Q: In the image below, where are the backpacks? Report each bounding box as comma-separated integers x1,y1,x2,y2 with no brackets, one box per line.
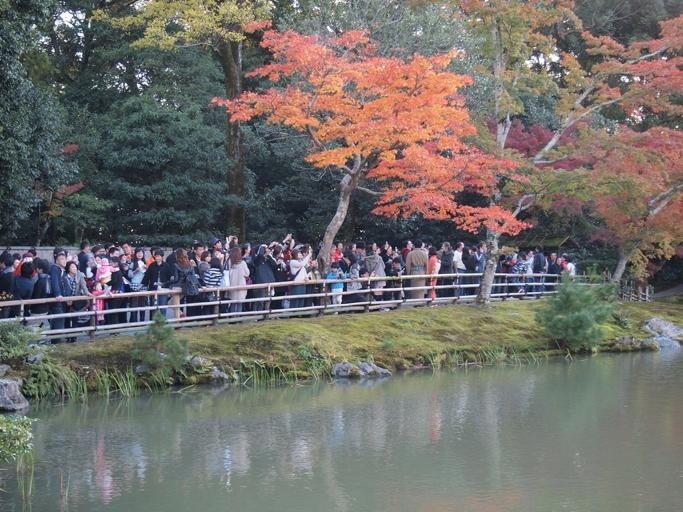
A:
174,262,199,298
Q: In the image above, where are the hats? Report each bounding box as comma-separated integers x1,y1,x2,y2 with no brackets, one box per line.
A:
291,248,302,259
207,237,221,247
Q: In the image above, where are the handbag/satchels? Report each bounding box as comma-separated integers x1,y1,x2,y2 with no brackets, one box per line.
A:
284,272,295,283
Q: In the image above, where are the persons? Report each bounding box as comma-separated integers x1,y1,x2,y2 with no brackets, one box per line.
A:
1,228,579,333
